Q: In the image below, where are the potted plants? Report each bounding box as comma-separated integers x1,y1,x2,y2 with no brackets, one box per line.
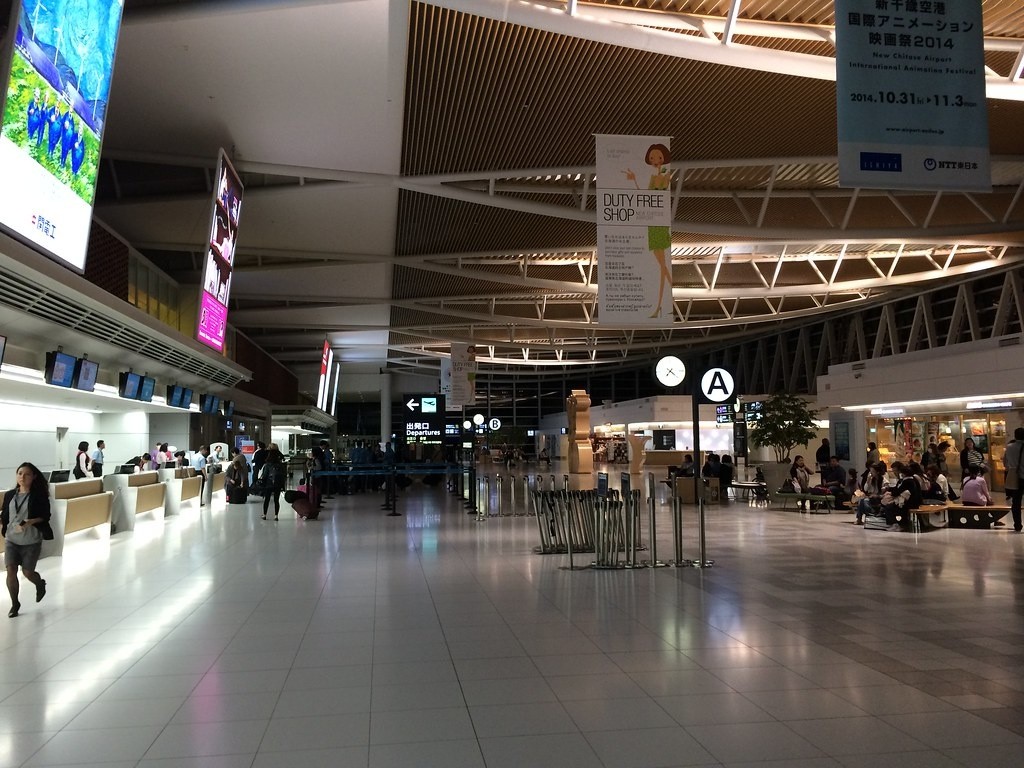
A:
755,395,821,501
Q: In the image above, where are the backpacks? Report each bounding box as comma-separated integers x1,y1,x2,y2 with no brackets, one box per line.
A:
915,474,946,504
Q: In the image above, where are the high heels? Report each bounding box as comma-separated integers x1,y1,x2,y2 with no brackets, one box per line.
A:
9,603,20,618
36,579,46,602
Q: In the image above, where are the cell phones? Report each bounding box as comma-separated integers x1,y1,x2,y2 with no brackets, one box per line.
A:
19,520,26,526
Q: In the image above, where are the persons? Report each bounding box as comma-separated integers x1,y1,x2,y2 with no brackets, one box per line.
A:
90,440,105,477
790,455,814,510
150,442,172,471
853,460,949,532
0,462,54,618
841,468,861,494
823,455,847,503
224,447,248,501
252,442,285,521
540,448,552,466
126,453,151,471
211,445,222,463
499,446,525,468
702,454,735,485
816,438,830,484
921,441,960,501
961,463,1005,526
1003,428,1024,531
681,454,693,474
306,440,332,504
867,442,879,462
192,445,208,506
349,440,395,493
73,441,91,479
960,438,984,477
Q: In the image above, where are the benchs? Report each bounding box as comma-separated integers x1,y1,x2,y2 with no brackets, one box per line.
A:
772,492,1024,532
729,478,766,489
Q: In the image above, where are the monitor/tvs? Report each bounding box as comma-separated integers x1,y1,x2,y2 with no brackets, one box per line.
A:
166,461,176,468
0,335,7,368
120,464,135,474
200,394,219,415
45,351,99,392
224,400,235,416
119,372,156,402
166,384,193,409
50,470,70,483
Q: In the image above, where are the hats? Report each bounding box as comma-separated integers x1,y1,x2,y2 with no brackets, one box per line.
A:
938,442,950,450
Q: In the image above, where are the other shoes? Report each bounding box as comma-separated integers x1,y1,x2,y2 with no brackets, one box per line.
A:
261,515,266,520
801,505,807,510
952,497,960,501
226,496,230,502
895,525,906,532
887,524,897,530
853,521,863,524
994,522,1005,526
274,518,278,521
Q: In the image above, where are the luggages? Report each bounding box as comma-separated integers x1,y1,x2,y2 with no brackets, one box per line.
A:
281,490,319,521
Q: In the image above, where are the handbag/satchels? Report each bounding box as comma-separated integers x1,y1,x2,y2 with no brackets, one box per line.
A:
256,479,266,487
779,478,801,494
809,485,833,495
230,485,247,504
